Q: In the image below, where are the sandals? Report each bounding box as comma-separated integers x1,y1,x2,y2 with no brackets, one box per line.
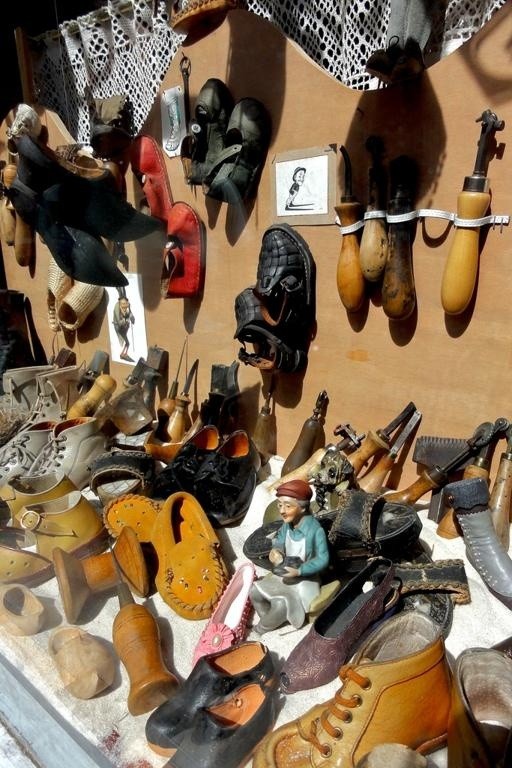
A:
276,555,400,691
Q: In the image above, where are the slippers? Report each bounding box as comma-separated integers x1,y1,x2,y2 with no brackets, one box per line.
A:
372,541,471,643
242,492,421,566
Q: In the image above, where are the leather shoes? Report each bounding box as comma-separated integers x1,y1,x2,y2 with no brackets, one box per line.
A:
255,222,318,345
166,682,274,766
145,640,275,756
238,609,456,760
232,290,281,377
1,367,263,623
2,77,273,331
445,644,512,760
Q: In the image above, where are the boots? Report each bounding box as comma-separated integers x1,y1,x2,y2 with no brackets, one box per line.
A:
449,475,512,603
366,0,446,88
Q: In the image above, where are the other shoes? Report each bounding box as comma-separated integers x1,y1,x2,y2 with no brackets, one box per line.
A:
49,626,115,700
190,560,259,668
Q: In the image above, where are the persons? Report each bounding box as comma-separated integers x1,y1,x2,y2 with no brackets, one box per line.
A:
248,480,330,640
112,286,135,362
284,167,314,210
